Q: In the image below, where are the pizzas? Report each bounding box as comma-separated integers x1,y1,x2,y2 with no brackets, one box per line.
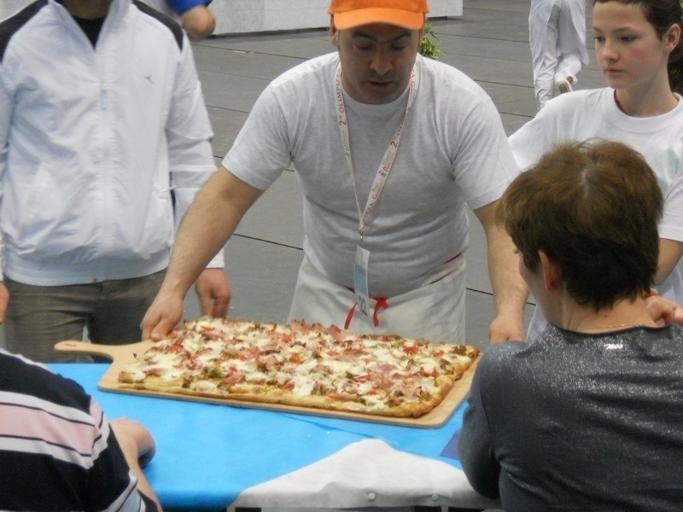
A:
118,315,480,418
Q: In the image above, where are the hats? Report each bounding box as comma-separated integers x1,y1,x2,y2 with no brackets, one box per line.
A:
327,0,429,32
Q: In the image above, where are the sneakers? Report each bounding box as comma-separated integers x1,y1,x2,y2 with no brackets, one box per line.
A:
557,78,574,93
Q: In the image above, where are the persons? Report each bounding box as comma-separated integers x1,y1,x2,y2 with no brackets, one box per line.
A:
520,0,590,113
136,0,528,349
0,1,235,370
2,272,163,512
448,136,680,509
495,1,681,349
